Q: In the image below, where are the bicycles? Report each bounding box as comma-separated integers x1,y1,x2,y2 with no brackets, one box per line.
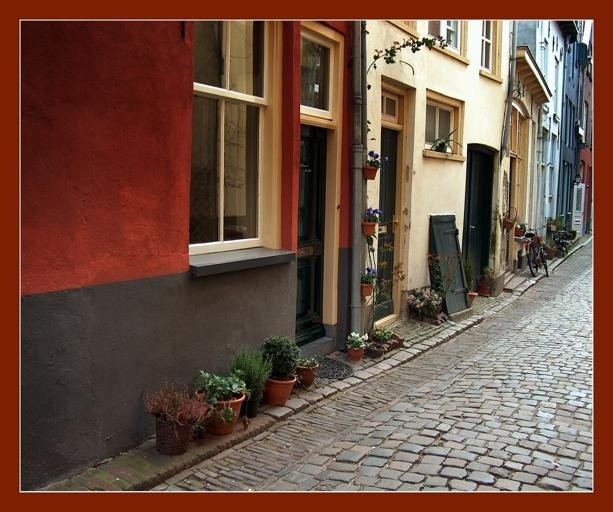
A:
557,223,570,258
523,225,549,277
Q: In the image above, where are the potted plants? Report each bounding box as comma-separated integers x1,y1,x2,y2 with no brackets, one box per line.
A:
464,263,478,305
430,128,463,155
548,209,565,231
143,337,319,455
546,240,556,260
478,264,494,296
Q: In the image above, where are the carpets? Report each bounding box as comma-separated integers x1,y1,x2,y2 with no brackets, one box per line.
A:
313,353,354,379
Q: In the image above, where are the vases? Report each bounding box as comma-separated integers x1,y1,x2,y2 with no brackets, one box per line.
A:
515,228,525,236
364,167,378,180
348,347,364,360
362,222,377,236
361,283,372,296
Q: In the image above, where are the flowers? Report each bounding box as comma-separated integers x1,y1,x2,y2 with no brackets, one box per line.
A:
361,267,375,284
515,224,526,230
408,288,442,308
362,208,382,222
364,150,389,169
345,332,369,350
